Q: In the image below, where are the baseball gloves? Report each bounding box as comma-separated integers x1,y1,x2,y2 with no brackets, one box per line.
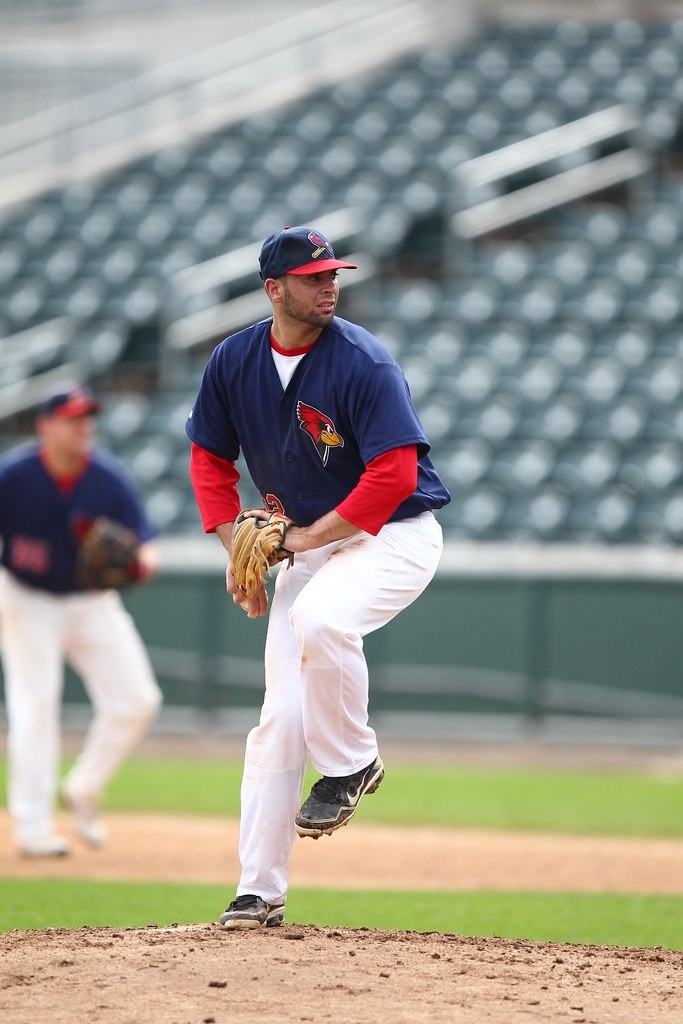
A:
229,507,295,620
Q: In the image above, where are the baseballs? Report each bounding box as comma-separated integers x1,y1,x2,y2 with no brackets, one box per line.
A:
239,587,269,613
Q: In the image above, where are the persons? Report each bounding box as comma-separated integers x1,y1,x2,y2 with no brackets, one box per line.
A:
185,226,452,929
0,388,164,859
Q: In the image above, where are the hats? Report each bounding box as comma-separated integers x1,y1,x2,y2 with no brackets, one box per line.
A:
258,225,357,281
37,381,98,419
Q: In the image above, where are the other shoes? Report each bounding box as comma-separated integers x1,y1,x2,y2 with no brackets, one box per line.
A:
18,836,68,857
56,784,101,846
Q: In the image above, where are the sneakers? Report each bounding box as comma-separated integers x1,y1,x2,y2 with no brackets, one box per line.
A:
293,754,384,838
219,895,285,930
